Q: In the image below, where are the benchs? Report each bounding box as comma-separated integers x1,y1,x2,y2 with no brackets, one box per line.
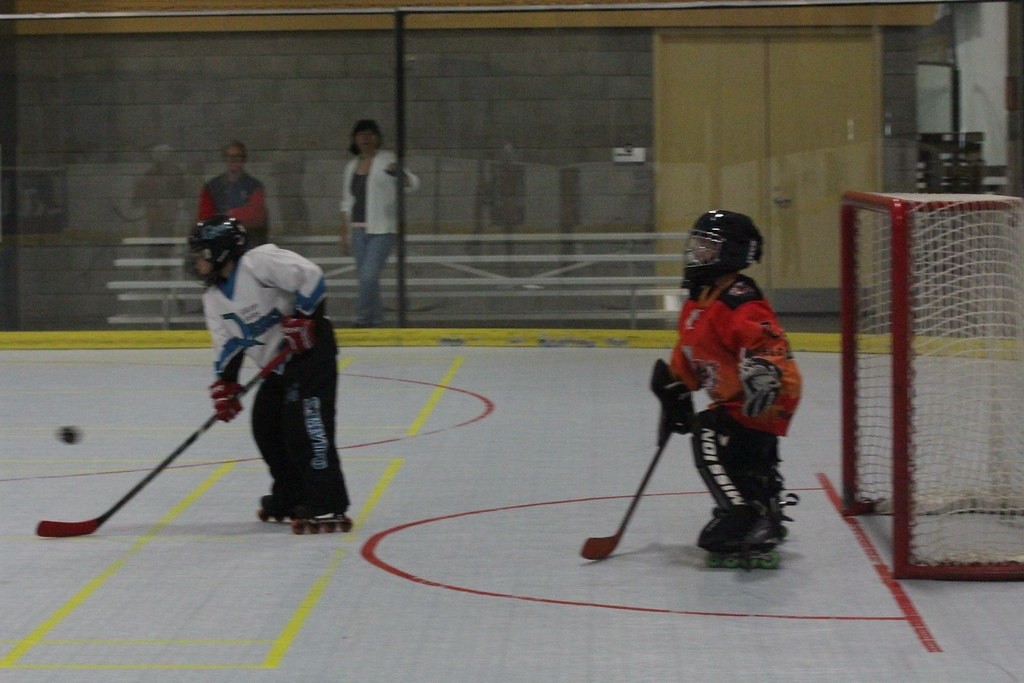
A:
110,229,690,321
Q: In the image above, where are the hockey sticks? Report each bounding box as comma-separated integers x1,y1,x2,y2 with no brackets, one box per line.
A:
580,415,677,561
36,346,291,538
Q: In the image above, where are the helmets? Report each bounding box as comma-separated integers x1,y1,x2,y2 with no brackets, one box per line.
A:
684,211,763,288
184,215,247,287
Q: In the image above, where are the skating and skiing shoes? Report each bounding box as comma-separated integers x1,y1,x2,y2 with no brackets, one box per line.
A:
288,503,352,535
698,496,788,570
257,485,294,522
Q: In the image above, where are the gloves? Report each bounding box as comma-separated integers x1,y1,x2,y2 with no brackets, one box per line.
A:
281,316,316,354
650,360,700,436
738,357,784,419
210,380,242,422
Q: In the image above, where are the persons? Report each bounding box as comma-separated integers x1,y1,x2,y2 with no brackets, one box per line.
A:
340,119,419,328
199,142,264,246
191,218,354,533
652,210,803,568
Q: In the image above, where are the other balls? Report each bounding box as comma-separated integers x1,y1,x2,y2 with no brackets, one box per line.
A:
62,427,76,444
